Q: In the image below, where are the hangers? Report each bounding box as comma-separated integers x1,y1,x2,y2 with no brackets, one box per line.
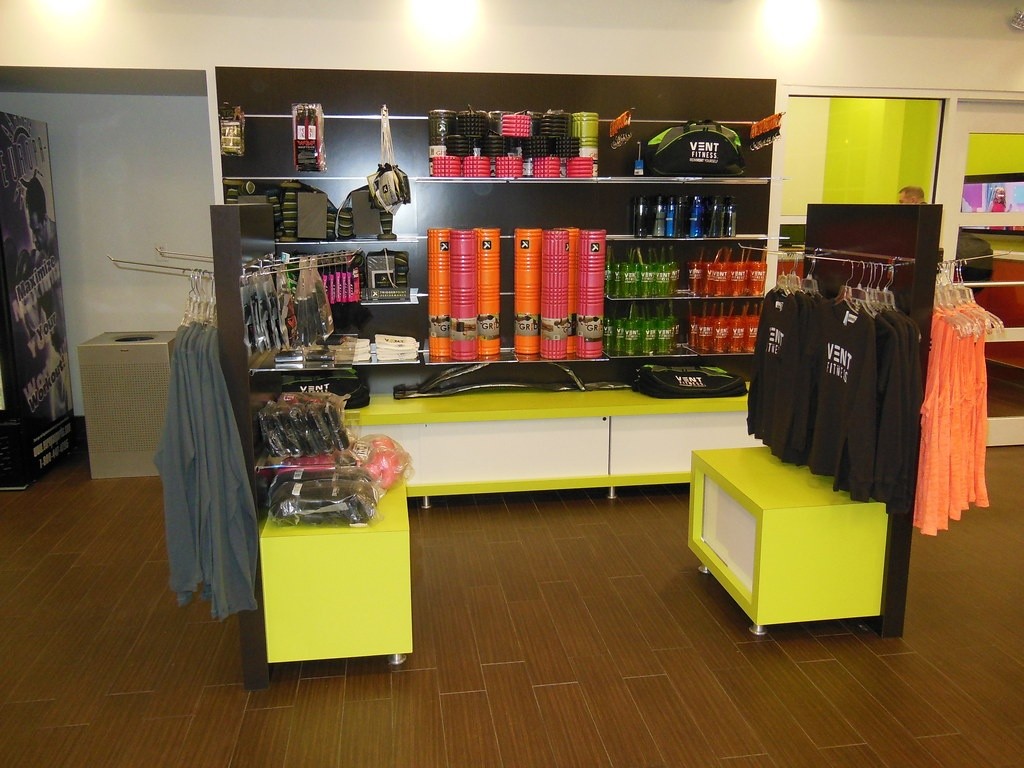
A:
934,258,1005,339
178,269,218,330
773,254,819,297
831,260,901,320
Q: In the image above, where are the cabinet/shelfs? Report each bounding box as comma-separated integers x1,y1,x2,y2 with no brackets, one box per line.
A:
688,446,889,635
259,476,414,664
216,65,776,509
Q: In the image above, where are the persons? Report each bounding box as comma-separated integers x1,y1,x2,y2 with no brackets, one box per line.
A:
23,177,74,354
898,185,925,204
983,187,1012,230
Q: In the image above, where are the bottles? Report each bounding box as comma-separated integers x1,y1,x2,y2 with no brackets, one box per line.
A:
635,195,737,237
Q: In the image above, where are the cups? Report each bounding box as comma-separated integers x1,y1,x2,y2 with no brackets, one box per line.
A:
604,317,760,354
605,259,767,297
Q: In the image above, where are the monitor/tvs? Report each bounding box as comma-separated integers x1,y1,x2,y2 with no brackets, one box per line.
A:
960,172,1024,236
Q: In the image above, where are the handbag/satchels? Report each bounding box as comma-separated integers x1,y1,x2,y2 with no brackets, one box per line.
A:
281,367,371,410
631,360,749,399
643,119,747,176
